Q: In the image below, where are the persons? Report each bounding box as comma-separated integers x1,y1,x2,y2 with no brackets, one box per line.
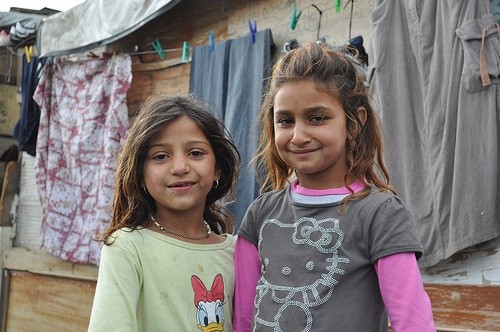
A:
233,41,438,332
86,94,237,332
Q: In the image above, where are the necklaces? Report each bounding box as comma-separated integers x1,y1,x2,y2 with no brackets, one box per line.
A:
150,214,212,240
